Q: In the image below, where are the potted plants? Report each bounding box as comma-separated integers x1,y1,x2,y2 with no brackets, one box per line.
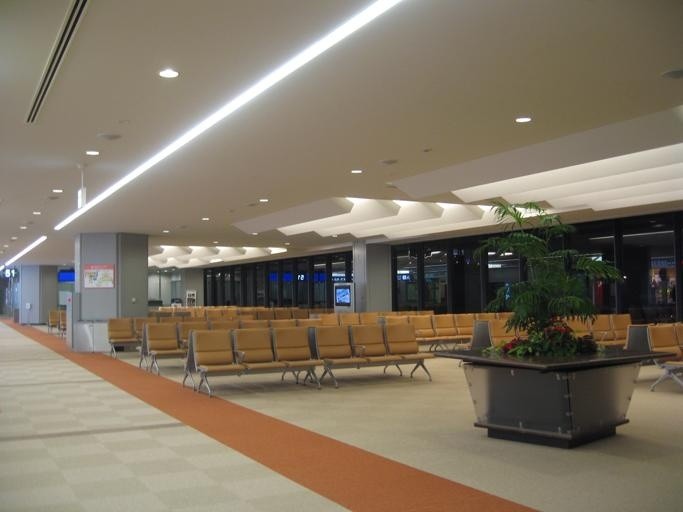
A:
433,200,680,448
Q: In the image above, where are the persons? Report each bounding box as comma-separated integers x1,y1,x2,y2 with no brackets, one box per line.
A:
651,268,673,304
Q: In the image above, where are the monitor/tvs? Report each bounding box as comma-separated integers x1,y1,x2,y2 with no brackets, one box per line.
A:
57,269,75,282
335,285,351,306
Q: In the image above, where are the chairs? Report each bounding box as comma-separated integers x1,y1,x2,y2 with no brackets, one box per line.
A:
48,306,683,397
